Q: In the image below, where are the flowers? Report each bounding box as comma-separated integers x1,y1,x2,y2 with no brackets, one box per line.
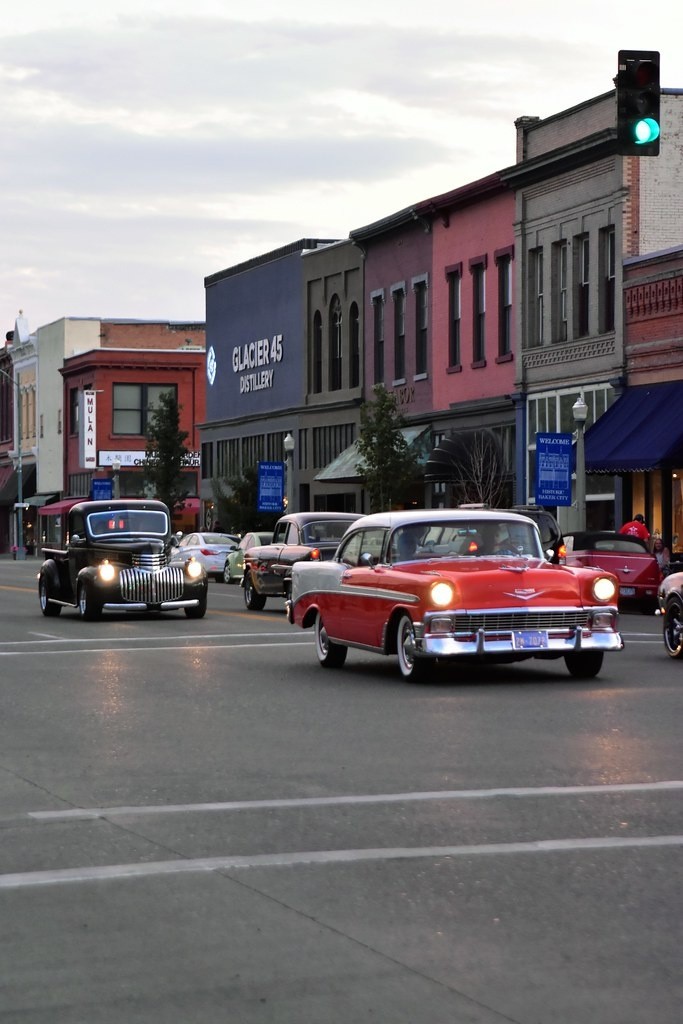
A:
10,546,27,552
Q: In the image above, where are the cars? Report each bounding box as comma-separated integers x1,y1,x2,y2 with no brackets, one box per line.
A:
283,508,625,681
37,497,209,622
170,530,243,584
239,510,395,611
657,572,683,658
221,531,315,588
562,529,665,615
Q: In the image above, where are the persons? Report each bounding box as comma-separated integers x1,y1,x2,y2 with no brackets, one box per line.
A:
213,521,225,533
397,533,418,561
618,514,670,567
463,523,515,556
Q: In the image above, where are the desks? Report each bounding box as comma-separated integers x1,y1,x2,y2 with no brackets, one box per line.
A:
665,562,683,575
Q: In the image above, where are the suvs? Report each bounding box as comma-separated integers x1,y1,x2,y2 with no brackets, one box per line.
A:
457,501,566,566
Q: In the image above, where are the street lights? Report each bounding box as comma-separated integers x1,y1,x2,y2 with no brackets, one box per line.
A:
571,394,589,531
283,434,297,515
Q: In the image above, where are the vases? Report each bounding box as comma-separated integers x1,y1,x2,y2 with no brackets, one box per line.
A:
12,550,29,560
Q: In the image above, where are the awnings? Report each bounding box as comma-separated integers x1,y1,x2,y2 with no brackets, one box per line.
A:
0,464,38,506
25,495,57,506
113,498,200,515
562,378,683,474
312,423,438,485
38,498,89,515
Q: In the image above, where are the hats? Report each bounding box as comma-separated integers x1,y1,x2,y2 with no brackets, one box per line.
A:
635,515,643,523
